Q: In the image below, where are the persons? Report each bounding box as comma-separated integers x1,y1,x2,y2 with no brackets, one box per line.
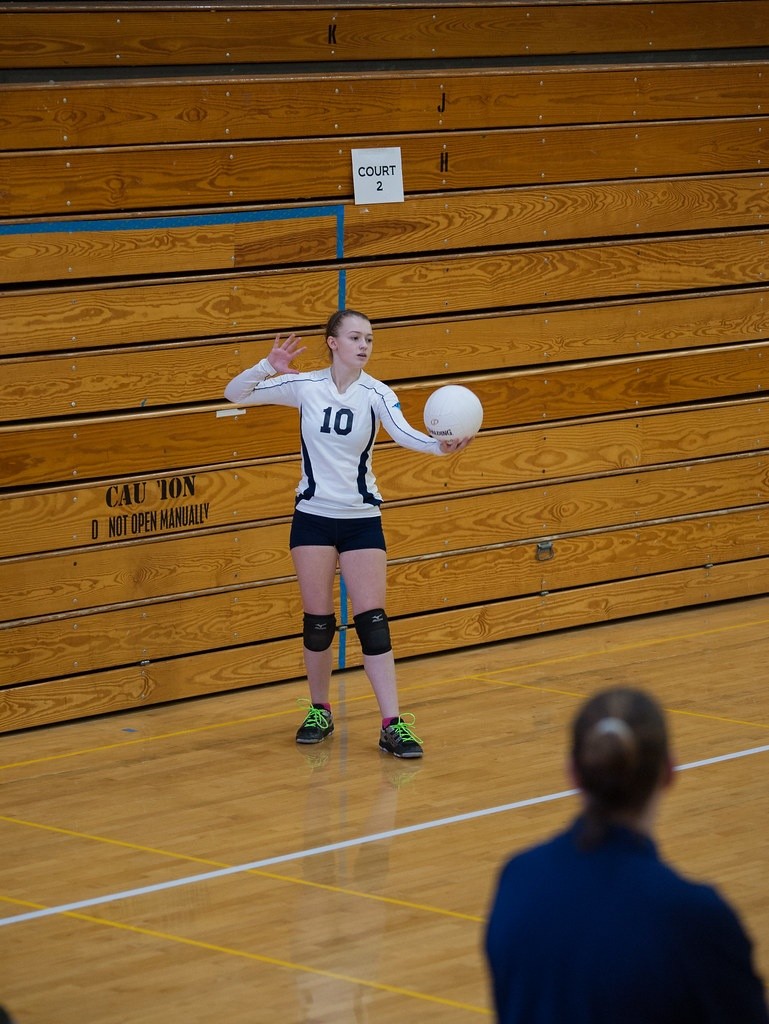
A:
222,307,478,762
479,683,767,1024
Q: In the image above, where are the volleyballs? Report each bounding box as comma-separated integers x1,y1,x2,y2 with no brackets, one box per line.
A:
423,385,484,446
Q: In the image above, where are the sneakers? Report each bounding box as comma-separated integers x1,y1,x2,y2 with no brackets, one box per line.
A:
296,708,335,744
379,717,423,759
